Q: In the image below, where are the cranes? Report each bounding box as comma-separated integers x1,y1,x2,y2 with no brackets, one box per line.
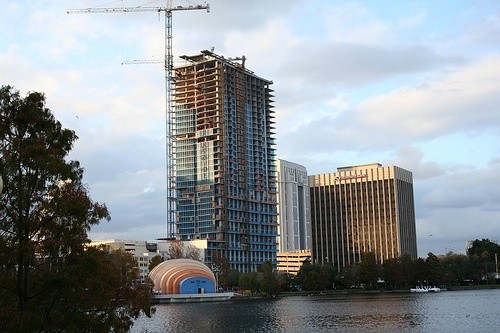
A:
67,0,210,245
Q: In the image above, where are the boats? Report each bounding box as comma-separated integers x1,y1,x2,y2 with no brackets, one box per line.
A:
410,279,440,293
